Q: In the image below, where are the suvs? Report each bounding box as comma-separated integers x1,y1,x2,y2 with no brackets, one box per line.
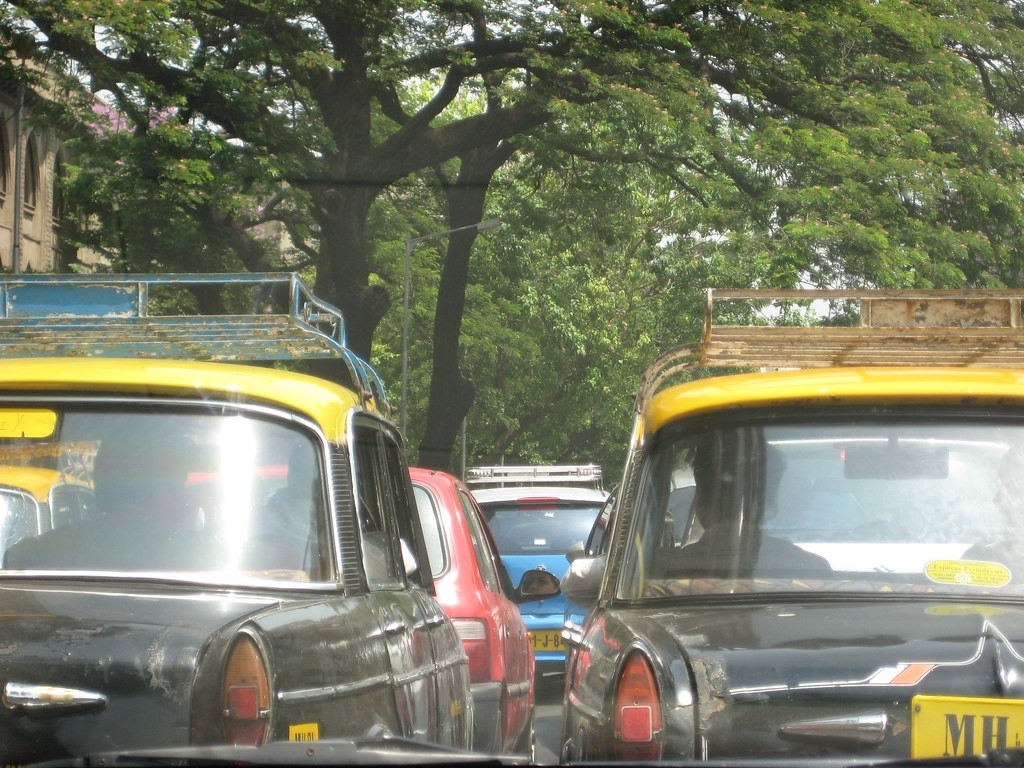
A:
567,288,1024,768
0,270,478,753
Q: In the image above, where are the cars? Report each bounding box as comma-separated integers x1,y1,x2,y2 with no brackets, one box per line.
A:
181,466,563,768
0,463,99,563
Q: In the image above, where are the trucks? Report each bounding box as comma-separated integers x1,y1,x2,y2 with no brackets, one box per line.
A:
462,463,622,702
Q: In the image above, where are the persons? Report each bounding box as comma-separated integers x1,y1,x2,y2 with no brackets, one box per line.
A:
523,571,558,594
251,440,417,578
962,438,1024,584
559,432,831,592
2,422,200,570
